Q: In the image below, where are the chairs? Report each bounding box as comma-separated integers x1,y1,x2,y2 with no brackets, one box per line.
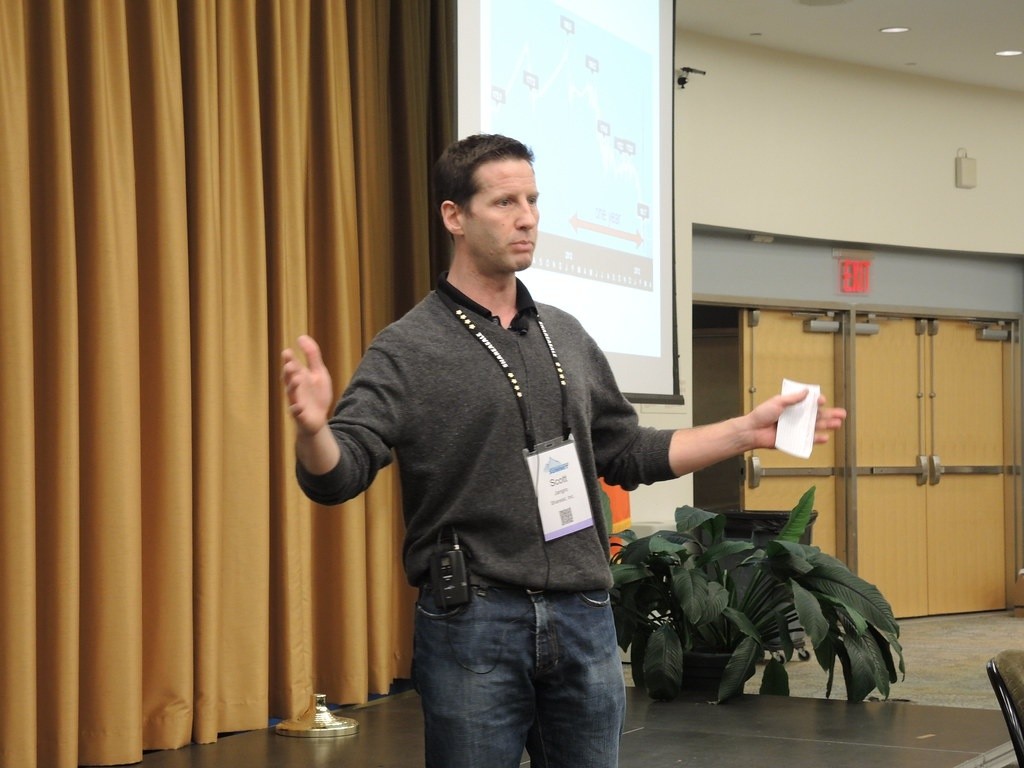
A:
987,649,1024,768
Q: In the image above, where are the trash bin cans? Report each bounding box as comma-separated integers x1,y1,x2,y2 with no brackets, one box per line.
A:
724,510,818,650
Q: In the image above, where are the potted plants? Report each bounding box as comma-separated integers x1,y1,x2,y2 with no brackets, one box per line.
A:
610,485,907,705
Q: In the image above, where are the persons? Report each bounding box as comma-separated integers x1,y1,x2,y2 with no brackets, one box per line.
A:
280,134,848,768
